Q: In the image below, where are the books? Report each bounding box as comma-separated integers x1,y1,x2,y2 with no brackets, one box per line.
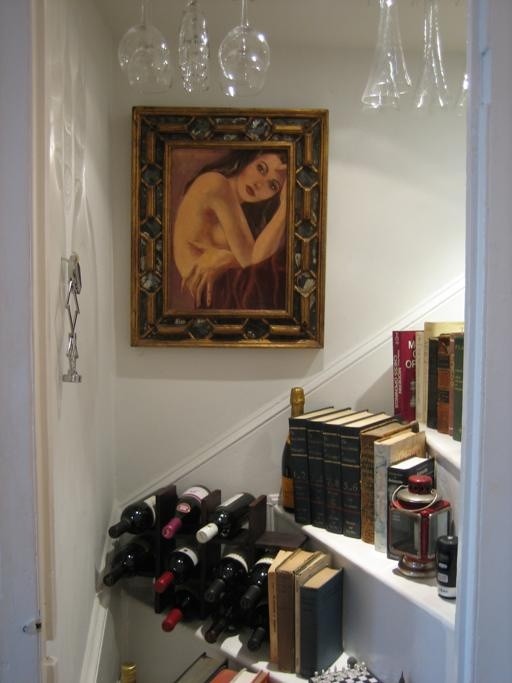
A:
374,428,427,553
360,420,419,544
307,408,370,529
386,454,435,560
268,549,294,670
275,548,332,674
342,413,400,538
289,405,351,523
300,566,344,681
322,411,385,533
393,323,462,441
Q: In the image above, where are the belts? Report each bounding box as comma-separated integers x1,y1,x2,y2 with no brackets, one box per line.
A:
216,1,271,99
117,1,172,93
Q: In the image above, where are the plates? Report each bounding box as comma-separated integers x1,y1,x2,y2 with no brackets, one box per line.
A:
118,402,465,682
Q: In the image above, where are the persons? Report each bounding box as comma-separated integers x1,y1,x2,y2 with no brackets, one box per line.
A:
172,150,287,312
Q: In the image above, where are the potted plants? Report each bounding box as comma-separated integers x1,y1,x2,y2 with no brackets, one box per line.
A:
125,103,328,355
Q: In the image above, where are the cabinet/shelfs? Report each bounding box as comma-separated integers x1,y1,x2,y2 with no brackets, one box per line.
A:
101,485,277,682
281,387,306,514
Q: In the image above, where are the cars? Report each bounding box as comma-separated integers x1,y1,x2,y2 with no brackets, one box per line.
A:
359,0,402,111
176,1,212,94
411,1,452,113
